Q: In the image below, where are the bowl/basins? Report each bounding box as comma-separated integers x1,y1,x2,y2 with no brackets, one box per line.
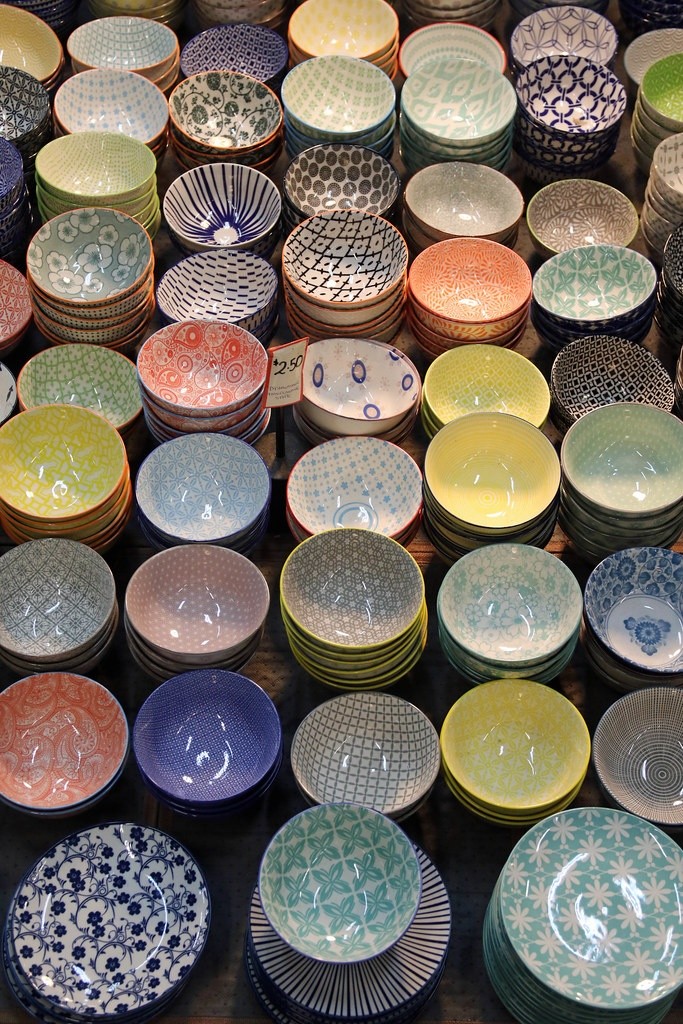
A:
0,0,683,962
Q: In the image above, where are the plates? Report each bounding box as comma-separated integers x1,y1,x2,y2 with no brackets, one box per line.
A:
0,822,211,1024
246,841,451,1024
483,806,683,1024
